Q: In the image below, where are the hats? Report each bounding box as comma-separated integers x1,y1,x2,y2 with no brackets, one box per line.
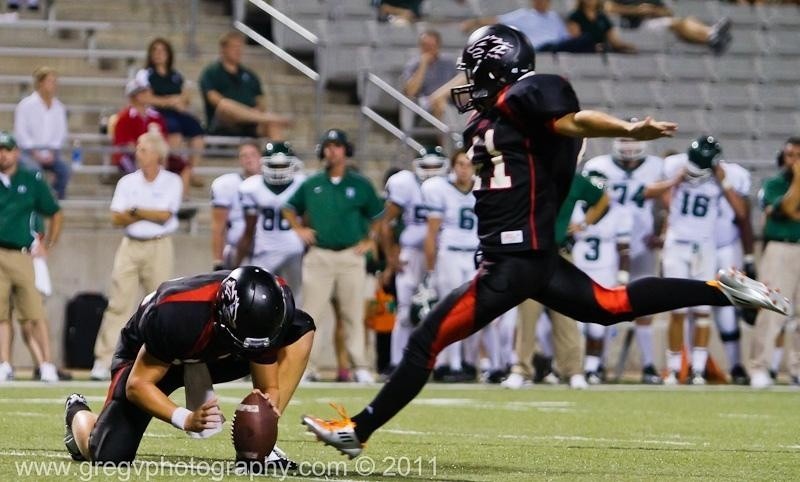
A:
316,128,354,158
0,133,25,152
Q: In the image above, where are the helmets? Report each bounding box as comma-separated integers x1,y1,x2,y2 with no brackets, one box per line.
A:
412,148,449,184
215,267,285,364
610,115,722,188
260,141,297,184
451,23,534,114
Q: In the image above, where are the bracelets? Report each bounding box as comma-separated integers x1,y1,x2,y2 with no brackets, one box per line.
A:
170,406,193,431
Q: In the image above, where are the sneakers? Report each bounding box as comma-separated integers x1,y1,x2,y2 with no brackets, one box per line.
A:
64,393,93,461
0,359,111,383
716,267,793,315
300,401,366,460
293,349,797,387
236,446,297,470
710,17,731,58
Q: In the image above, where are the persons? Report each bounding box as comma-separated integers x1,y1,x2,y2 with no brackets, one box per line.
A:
60,262,319,473
301,24,795,463
1,1,799,385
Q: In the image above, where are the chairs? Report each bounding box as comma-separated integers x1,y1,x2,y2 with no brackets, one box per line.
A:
271,1,800,239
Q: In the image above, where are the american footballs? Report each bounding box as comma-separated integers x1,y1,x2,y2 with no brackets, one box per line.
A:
231,393,278,472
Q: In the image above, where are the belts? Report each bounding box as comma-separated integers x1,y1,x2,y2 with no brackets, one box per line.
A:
767,236,800,244
0,243,30,253
447,246,477,253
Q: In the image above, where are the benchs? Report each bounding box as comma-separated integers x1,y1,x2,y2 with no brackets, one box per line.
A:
0,1,419,236
